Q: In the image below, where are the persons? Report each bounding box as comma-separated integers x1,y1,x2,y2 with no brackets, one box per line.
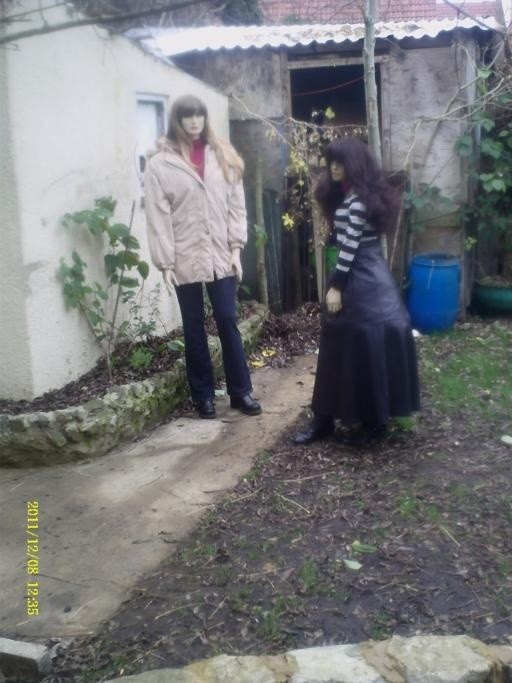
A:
142,95,262,416
295,138,421,444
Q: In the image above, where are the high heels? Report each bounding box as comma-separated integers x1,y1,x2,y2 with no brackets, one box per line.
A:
197,400,216,418
230,394,262,415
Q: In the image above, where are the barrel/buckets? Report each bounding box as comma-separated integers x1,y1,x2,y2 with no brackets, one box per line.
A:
407,249,462,337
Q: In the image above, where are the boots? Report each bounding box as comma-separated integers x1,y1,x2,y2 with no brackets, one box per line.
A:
294,416,335,445
345,420,387,446
